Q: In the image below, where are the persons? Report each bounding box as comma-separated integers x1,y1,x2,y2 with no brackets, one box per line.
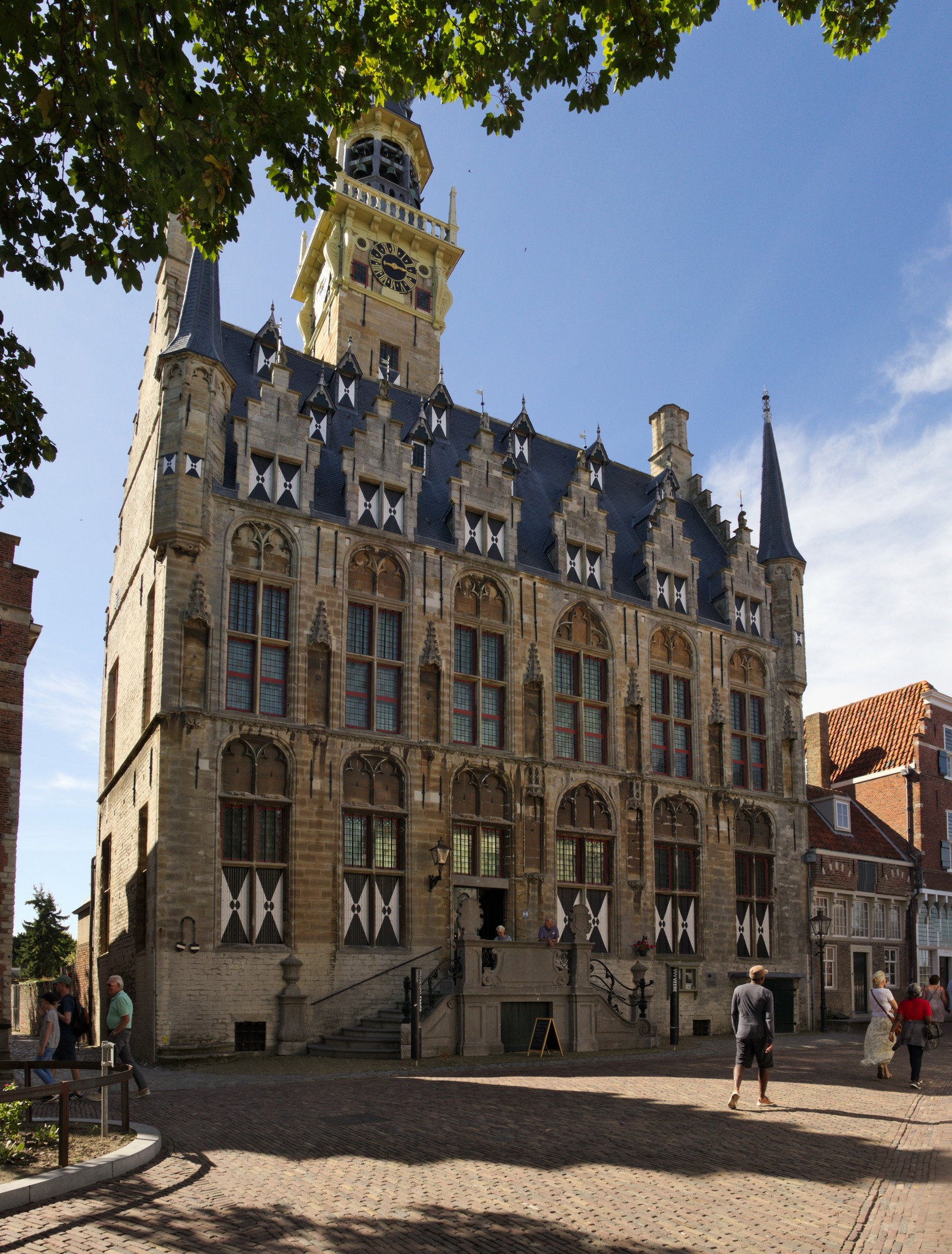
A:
922,974,949,1046
860,970,898,1079
36,974,83,1101
494,925,512,940
728,965,774,1110
538,915,559,947
34,992,59,1105
945,980,952,1014
888,983,932,1089
87,975,150,1101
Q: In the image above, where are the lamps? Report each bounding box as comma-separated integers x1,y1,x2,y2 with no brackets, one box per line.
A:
429,837,452,892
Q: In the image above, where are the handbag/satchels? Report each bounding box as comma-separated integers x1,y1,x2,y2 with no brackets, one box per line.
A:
890,1016,902,1036
926,1021,944,1050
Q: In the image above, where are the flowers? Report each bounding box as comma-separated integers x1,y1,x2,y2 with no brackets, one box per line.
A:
633,938,656,952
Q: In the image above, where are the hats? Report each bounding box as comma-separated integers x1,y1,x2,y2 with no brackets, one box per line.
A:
50,975,72,985
37,992,57,1002
749,965,769,979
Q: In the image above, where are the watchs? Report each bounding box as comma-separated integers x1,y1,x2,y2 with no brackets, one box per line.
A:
111,1031,116,1035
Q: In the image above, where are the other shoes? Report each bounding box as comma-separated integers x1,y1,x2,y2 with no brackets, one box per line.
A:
129,1088,151,1099
756,1095,774,1106
916,1077,922,1085
68,1091,83,1101
46,1094,59,1102
728,1088,740,1109
35,1096,45,1100
86,1092,102,1101
910,1081,921,1089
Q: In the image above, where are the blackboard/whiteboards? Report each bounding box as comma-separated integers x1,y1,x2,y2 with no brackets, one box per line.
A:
528,1017,562,1053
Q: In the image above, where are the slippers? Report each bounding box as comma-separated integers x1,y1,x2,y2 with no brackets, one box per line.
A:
884,1072,892,1080
877,1065,884,1079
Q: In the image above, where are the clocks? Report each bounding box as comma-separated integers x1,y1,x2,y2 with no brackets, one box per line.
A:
370,243,418,294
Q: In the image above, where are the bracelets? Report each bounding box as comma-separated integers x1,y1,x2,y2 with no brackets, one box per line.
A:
889,1032,893,1034
552,939,554,942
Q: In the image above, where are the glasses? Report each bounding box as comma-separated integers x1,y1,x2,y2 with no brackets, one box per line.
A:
106,983,117,987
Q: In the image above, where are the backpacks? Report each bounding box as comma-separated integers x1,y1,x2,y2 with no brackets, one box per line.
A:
59,995,90,1035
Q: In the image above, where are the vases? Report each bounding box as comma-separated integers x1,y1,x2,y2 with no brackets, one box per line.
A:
639,951,647,957
